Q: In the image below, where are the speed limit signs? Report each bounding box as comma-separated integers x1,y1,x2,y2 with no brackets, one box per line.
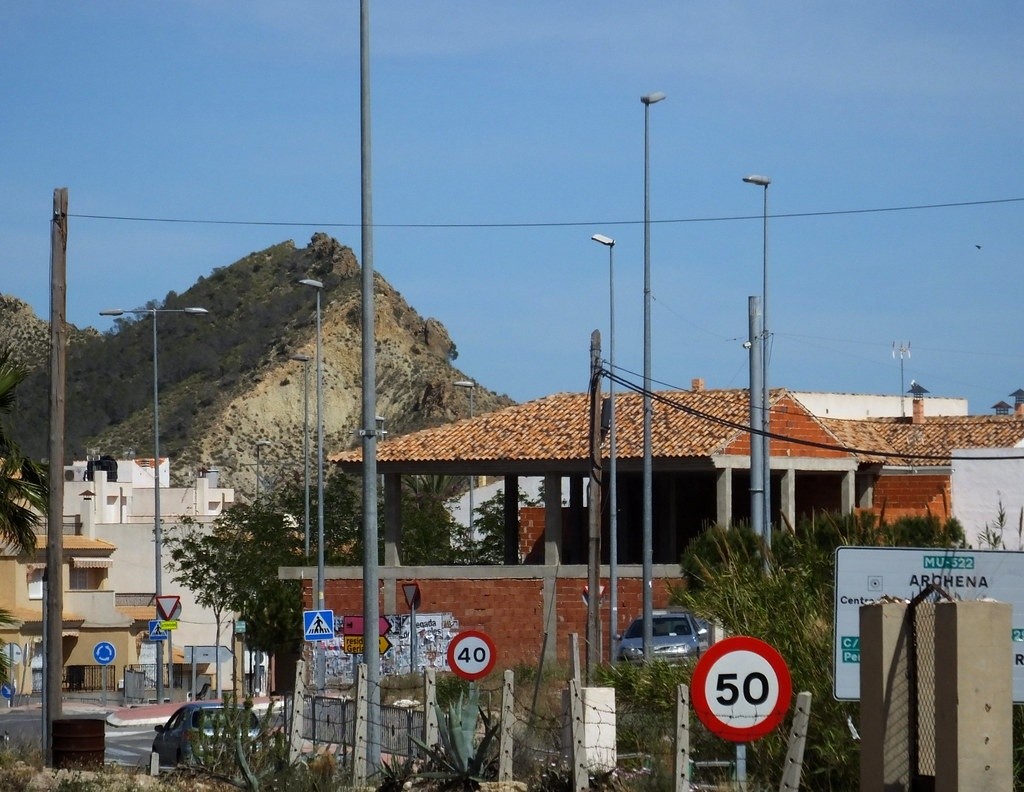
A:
692,636,793,743
447,630,496,680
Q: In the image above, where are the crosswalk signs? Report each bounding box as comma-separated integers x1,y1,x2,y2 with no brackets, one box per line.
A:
148,619,168,641
303,609,335,641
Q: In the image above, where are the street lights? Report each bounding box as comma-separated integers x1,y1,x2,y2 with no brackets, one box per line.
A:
292,354,310,568
98,307,209,703
376,415,387,501
453,380,476,561
741,173,775,590
591,232,620,687
256,440,268,501
639,91,672,667
298,276,323,691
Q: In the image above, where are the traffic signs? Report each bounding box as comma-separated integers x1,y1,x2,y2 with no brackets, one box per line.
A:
344,616,392,636
344,636,393,655
832,545,1024,703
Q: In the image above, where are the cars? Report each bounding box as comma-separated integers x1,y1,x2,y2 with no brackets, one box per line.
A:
154,701,264,767
614,610,709,661
